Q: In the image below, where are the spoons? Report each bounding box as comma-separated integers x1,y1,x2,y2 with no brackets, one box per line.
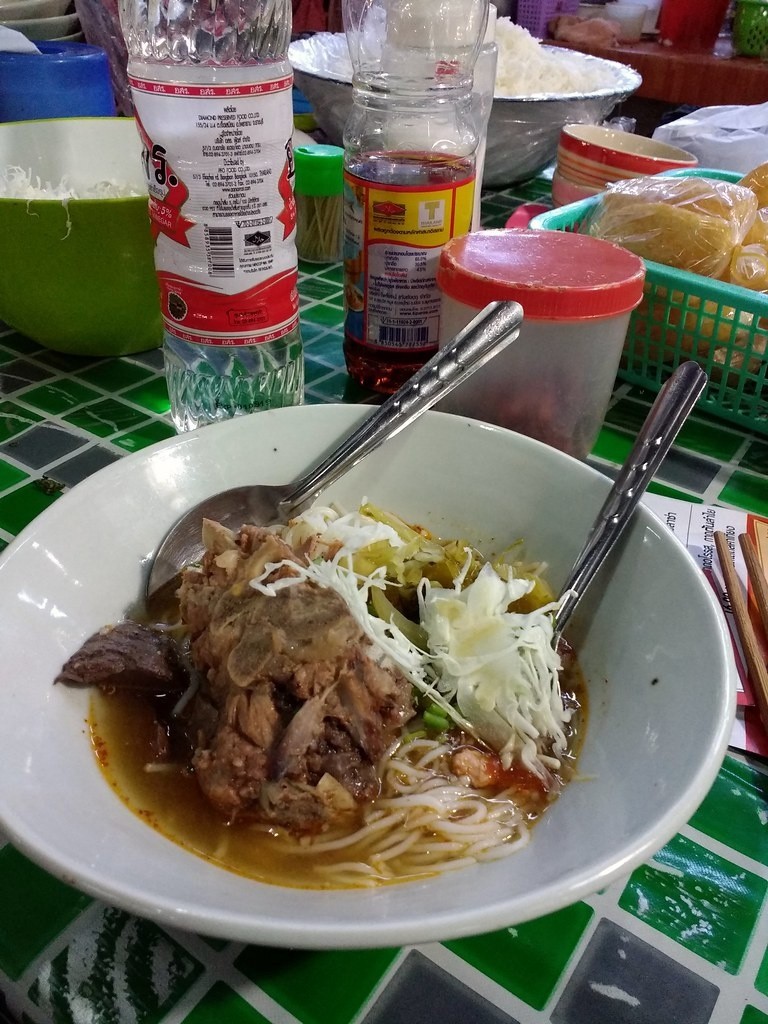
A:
145,301,524,627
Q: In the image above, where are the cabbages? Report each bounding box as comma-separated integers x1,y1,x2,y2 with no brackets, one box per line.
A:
246,505,581,785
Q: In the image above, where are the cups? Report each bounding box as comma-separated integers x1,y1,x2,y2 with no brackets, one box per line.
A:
656,0,730,54
605,3,646,42
437,229,645,462
619,0,662,30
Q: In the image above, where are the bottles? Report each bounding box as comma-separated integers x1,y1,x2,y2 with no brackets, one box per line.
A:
119,0,304,433
294,144,344,265
343,0,490,393
470,3,498,233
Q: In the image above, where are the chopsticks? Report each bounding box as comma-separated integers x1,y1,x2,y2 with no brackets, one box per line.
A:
714,531,768,722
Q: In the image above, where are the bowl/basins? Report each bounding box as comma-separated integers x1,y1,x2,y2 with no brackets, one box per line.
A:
551,169,606,235
0,117,166,357
0,403,737,950
289,33,643,185
0,0,85,42
557,123,697,189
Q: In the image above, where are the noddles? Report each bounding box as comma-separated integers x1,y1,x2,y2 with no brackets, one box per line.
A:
55,504,590,893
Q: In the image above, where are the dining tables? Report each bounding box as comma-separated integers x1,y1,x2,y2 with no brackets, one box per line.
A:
4,252,768,1024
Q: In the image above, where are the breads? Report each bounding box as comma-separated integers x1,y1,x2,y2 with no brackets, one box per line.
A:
584,163,768,384
